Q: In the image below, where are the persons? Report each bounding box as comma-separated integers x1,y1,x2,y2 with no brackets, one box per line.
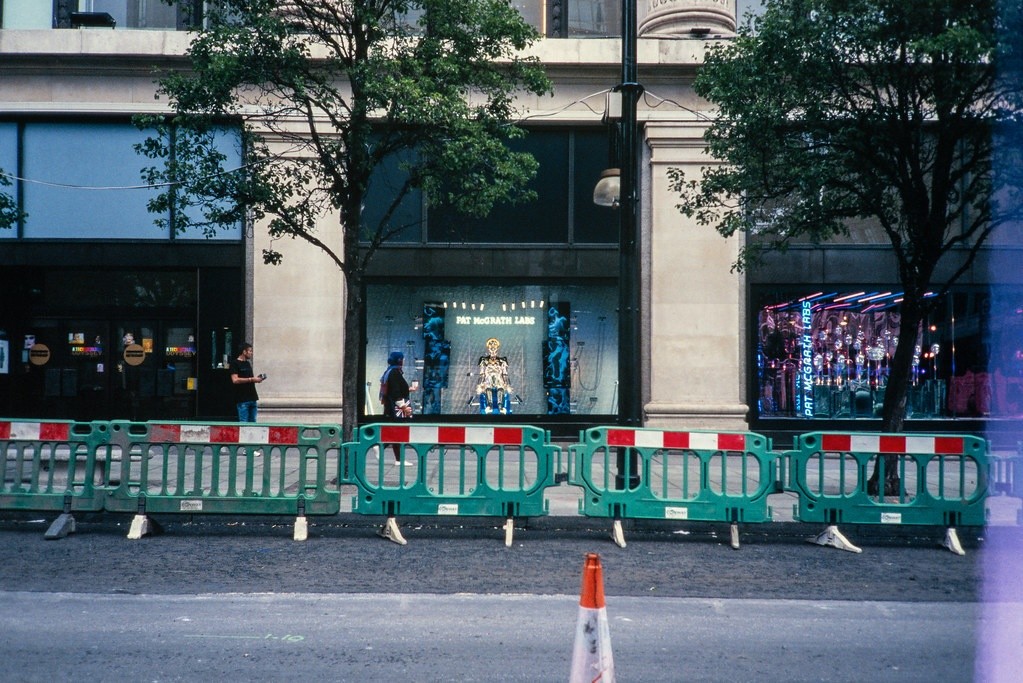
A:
473,338,516,415
220,343,268,457
373,352,418,467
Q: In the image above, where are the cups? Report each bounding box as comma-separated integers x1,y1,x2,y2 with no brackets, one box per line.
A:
412,381,418,390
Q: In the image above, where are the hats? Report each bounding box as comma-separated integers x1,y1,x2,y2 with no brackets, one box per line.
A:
387,352,403,366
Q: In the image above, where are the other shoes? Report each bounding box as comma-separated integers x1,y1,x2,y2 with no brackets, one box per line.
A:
220,446,229,452
373,445,380,461
395,459,413,467
243,449,260,457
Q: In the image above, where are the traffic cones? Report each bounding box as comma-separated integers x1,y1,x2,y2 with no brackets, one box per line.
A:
568,553,616,683
